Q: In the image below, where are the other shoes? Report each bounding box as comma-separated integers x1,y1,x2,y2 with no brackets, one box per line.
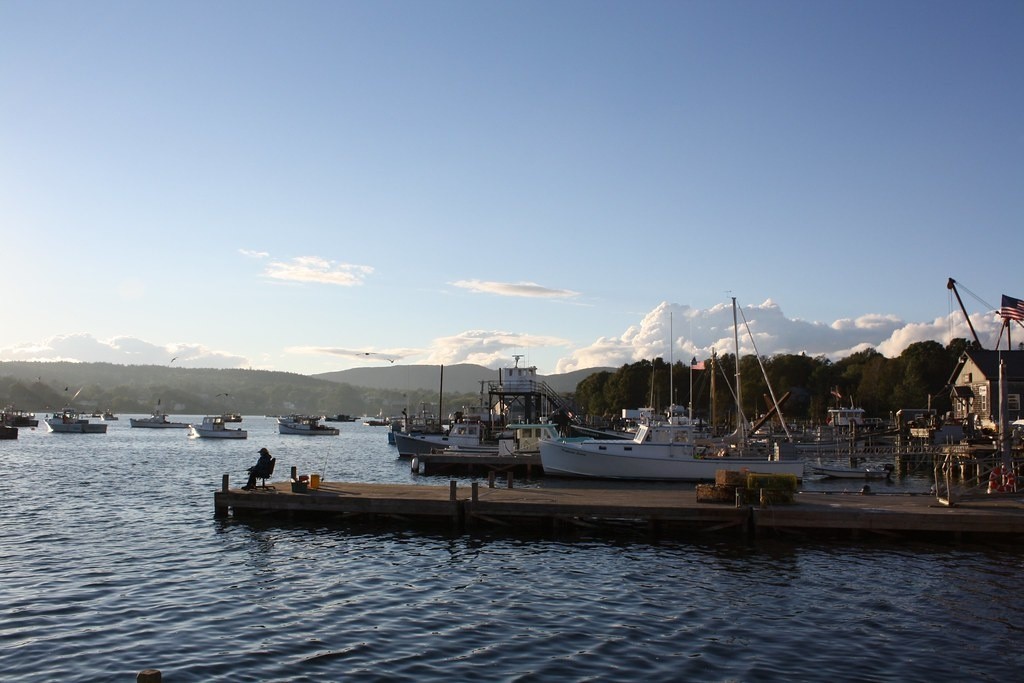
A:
241,485,256,490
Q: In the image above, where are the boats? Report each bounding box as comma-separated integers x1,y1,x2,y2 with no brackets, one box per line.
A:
538,295,984,484
49,415,107,434
44,383,102,428
277,413,317,425
219,412,242,422
324,414,361,422
194,417,248,440
129,413,192,428
103,409,118,421
366,416,393,428
0,400,39,441
395,355,607,474
388,365,468,445
278,417,339,435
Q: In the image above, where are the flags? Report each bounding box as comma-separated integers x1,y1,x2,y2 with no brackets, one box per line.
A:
1001,293,1024,322
691,359,706,370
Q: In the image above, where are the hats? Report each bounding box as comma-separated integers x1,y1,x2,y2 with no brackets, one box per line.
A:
256,448,267,453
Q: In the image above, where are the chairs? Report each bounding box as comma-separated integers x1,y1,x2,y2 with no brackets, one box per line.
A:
247,457,276,491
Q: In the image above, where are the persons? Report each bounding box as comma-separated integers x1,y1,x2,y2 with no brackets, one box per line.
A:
241,446,274,490
477,419,487,445
